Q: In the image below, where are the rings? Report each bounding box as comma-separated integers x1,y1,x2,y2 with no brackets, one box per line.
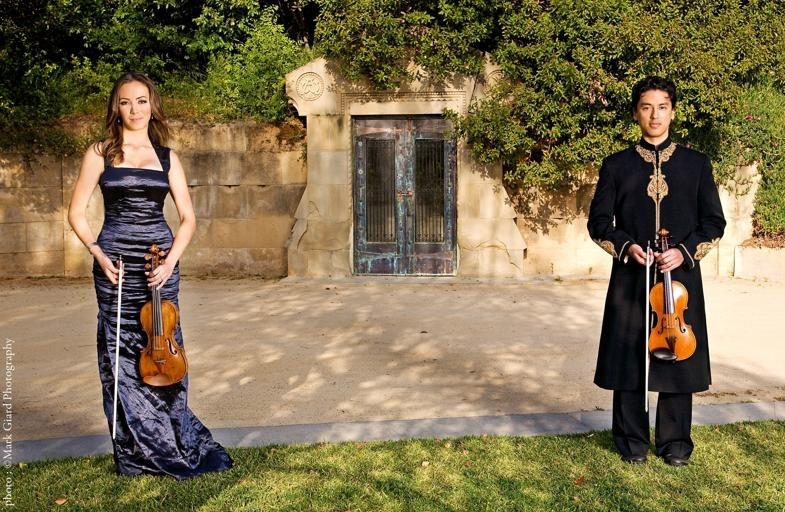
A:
160,278,164,282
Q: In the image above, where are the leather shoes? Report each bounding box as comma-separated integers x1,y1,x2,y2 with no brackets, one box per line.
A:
665,452,689,466
621,454,647,462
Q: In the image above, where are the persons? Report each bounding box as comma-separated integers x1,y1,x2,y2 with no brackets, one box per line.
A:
68,73,233,481
587,76,727,466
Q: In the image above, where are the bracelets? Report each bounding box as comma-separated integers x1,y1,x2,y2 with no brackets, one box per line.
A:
87,242,98,254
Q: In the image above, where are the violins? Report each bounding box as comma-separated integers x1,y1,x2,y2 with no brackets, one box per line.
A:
648,229,697,362
139,243,187,387
